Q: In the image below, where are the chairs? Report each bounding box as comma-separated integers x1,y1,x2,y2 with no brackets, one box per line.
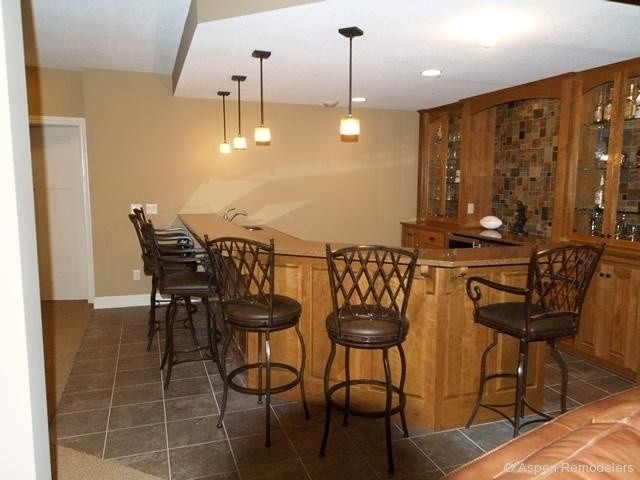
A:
462,239,609,443
123,204,225,393
316,241,411,474
201,228,311,449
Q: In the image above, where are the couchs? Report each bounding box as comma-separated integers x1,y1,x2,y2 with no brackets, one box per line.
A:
422,382,640,479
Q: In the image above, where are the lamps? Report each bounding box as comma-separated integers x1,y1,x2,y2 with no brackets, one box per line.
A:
231,75,247,149
337,27,365,136
251,49,272,144
217,91,231,154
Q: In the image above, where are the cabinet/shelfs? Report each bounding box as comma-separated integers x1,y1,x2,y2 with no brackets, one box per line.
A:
561,235,640,383
399,222,486,252
562,58,639,243
416,94,494,228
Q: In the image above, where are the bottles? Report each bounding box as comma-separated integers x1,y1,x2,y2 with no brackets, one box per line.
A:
593,175,606,210
623,84,637,120
592,125,609,169
593,90,605,123
604,88,614,122
